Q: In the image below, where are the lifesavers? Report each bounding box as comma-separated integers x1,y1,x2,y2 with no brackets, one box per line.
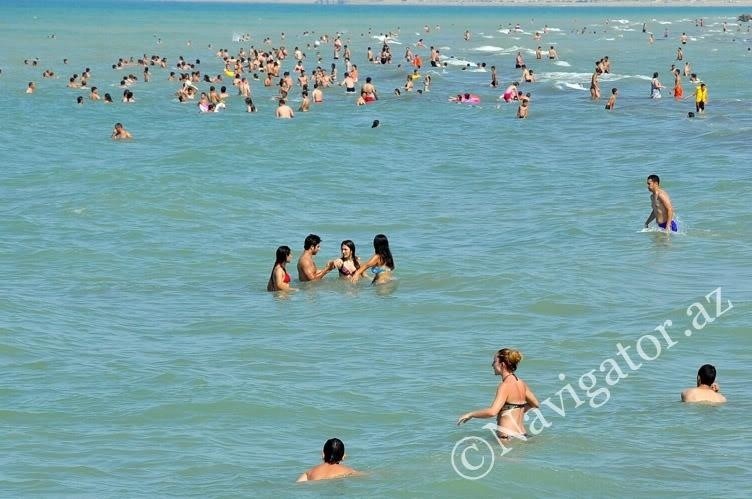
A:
225,70,232,78
461,92,480,105
412,72,421,79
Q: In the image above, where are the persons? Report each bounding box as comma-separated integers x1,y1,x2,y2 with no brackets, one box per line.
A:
267,231,394,295
458,349,539,442
293,436,364,483
682,363,726,405
1,14,752,142
644,175,678,233
